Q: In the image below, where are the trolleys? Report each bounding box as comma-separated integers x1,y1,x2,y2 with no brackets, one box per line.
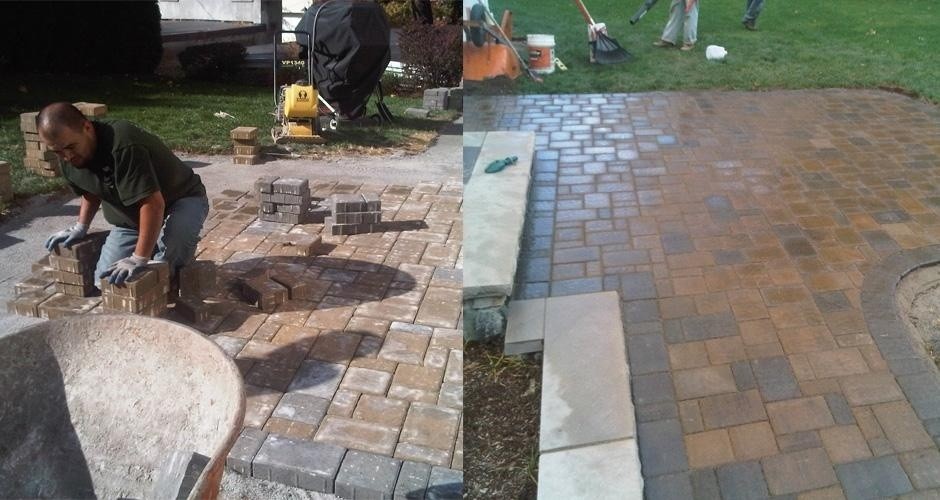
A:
271,29,329,147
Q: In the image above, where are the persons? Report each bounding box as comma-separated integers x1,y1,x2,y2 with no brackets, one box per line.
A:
35,101,211,290
463,0,495,43
653,0,701,51
741,0,766,32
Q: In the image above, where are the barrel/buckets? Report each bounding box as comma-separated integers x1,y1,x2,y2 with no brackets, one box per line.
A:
705,44,728,59
527,32,557,75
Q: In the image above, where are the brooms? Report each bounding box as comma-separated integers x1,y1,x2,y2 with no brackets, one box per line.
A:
575,0,633,65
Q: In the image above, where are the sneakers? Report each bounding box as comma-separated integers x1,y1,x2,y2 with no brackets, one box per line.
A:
680,43,696,51
742,19,757,31
653,40,674,48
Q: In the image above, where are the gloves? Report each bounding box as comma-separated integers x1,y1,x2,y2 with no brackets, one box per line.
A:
98,252,149,286
44,222,89,252
